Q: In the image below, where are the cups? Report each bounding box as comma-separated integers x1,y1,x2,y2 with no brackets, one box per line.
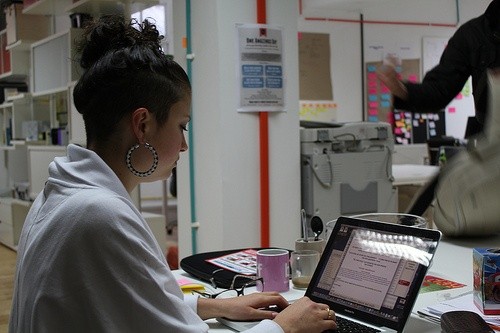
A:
255,248,290,293
290,249,319,290
294,236,325,255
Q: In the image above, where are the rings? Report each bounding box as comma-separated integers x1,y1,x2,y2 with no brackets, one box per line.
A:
324,307,331,319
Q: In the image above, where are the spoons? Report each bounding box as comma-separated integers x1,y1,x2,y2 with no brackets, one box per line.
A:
310,215,324,241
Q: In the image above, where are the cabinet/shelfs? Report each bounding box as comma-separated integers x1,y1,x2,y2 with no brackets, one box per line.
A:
0,0,173,255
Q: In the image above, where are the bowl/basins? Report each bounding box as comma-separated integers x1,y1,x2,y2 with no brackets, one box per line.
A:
325,213,428,247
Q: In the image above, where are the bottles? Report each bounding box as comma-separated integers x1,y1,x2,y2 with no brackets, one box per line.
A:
439,146,447,173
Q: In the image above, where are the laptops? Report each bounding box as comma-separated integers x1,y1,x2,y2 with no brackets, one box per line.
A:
215,216,443,333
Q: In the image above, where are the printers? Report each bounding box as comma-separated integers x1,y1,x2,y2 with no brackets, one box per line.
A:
300,119,399,237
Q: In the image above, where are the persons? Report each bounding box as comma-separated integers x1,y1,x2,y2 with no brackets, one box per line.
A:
7,14,337,333
373,0,500,151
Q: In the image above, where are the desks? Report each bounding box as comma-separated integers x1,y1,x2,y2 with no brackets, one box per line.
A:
171,242,481,333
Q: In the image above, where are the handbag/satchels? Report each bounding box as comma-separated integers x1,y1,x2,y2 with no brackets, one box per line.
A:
180,247,300,291
